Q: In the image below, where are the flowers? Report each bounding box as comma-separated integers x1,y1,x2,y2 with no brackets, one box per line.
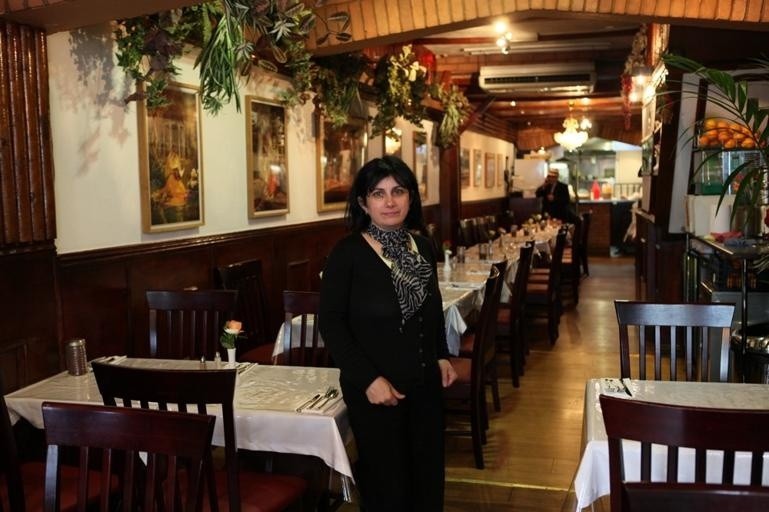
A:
442,239,451,249
220,319,242,348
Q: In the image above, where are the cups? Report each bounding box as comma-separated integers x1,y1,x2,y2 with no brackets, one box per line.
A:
453,210,562,264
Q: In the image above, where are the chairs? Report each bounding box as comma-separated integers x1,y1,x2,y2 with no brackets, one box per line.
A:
145,287,241,360
41,400,216,511
443,265,503,467
454,210,590,347
285,257,314,291
611,296,736,383
598,391,769,511
460,253,509,429
680,225,769,383
498,243,533,389
206,256,278,365
90,361,308,510
277,289,338,364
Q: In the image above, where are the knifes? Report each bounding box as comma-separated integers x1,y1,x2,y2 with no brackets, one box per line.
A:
619,377,633,396
296,394,320,411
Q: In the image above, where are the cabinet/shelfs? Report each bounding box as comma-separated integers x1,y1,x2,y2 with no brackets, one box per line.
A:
640,80,666,177
688,146,769,195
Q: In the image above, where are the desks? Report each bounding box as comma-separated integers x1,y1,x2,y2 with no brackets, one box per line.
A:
435,271,488,313
572,374,768,512
272,282,478,359
4,354,359,511
438,260,513,302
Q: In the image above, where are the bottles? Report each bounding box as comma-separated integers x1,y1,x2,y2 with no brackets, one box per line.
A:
63,337,89,377
592,177,600,199
213,351,222,370
503,154,510,197
200,355,207,370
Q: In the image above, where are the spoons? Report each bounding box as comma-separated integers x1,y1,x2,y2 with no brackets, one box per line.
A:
608,377,622,392
317,389,340,412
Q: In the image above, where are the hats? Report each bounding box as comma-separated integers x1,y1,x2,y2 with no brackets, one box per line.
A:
548,169,559,176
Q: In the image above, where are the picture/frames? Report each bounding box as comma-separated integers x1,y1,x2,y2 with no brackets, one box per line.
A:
135,75,206,233
315,107,369,216
243,94,292,218
382,125,512,202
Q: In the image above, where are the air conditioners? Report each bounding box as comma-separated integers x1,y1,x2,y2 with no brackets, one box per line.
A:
479,64,598,99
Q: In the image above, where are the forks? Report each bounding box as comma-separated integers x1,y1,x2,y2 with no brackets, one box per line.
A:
307,386,334,408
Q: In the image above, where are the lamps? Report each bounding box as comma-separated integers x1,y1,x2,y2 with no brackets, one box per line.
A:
554,101,589,154
493,19,514,56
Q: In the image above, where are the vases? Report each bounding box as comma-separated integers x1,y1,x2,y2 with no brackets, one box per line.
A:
225,348,241,387
443,251,453,279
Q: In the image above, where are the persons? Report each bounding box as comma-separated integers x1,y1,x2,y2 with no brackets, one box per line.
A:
535,169,571,221
316,157,460,511
